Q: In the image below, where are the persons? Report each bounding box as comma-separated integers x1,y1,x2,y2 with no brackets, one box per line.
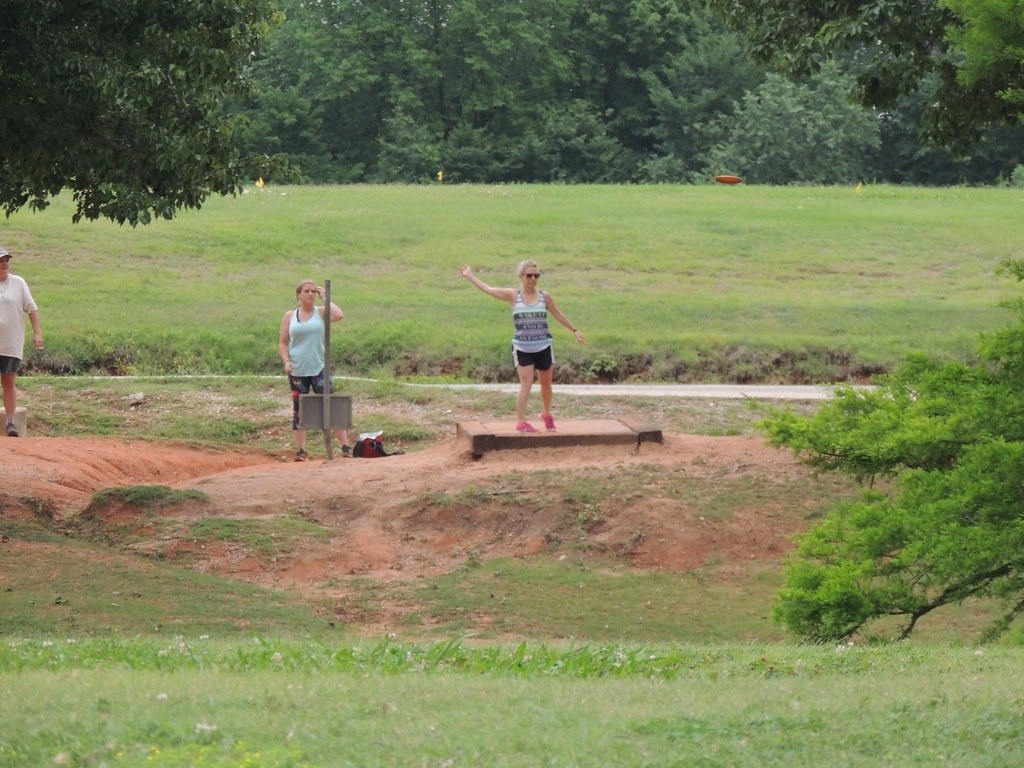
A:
280,281,353,462
0,249,42,437
460,260,585,433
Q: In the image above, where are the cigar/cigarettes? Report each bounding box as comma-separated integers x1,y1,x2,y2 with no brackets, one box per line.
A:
34,347,43,349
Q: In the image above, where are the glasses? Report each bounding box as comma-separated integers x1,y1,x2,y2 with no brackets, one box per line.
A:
523,273,541,279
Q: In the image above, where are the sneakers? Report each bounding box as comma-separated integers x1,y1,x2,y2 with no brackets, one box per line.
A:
5,422,18,436
295,449,308,461
542,413,557,432
515,421,539,434
342,444,351,456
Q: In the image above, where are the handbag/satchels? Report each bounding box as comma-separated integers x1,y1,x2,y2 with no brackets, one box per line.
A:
353,431,404,458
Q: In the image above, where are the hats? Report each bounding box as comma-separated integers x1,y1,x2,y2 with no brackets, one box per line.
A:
0,246,12,259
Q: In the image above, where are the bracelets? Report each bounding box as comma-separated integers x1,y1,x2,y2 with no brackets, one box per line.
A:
573,329,578,332
284,359,290,364
